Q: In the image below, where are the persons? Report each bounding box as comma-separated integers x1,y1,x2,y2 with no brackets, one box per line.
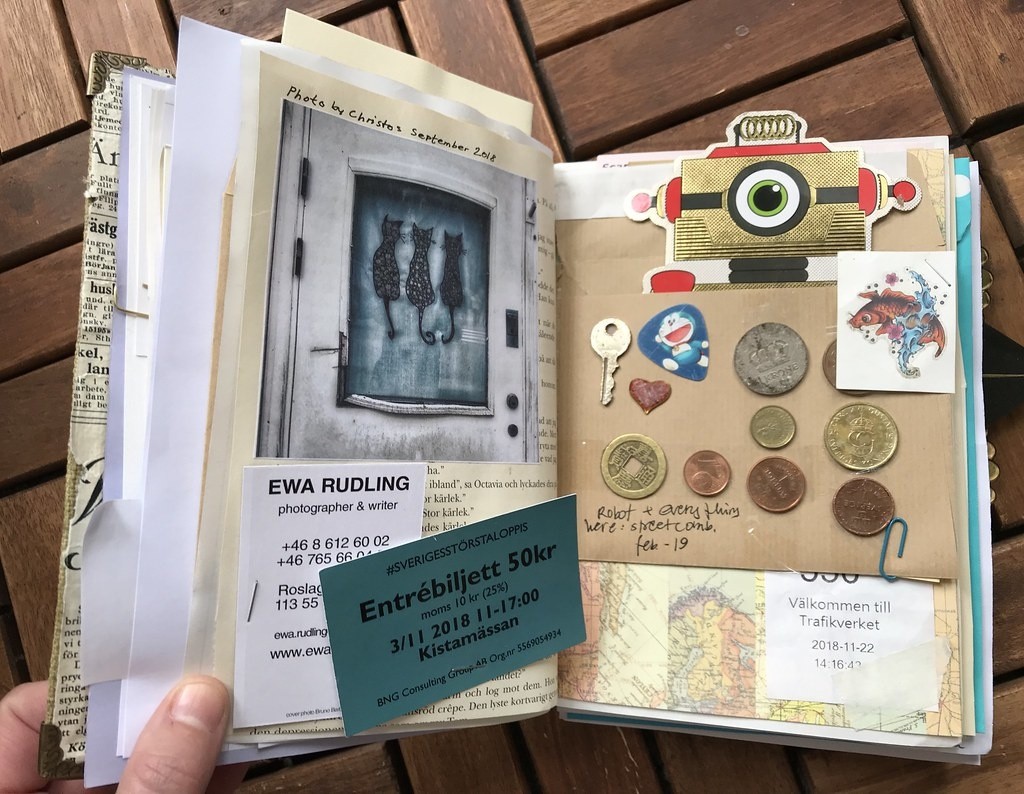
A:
0,676,231,794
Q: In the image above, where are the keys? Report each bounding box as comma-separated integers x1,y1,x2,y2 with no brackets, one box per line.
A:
589,317,631,406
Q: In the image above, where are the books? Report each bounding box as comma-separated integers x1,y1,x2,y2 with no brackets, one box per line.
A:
39,12,996,771
